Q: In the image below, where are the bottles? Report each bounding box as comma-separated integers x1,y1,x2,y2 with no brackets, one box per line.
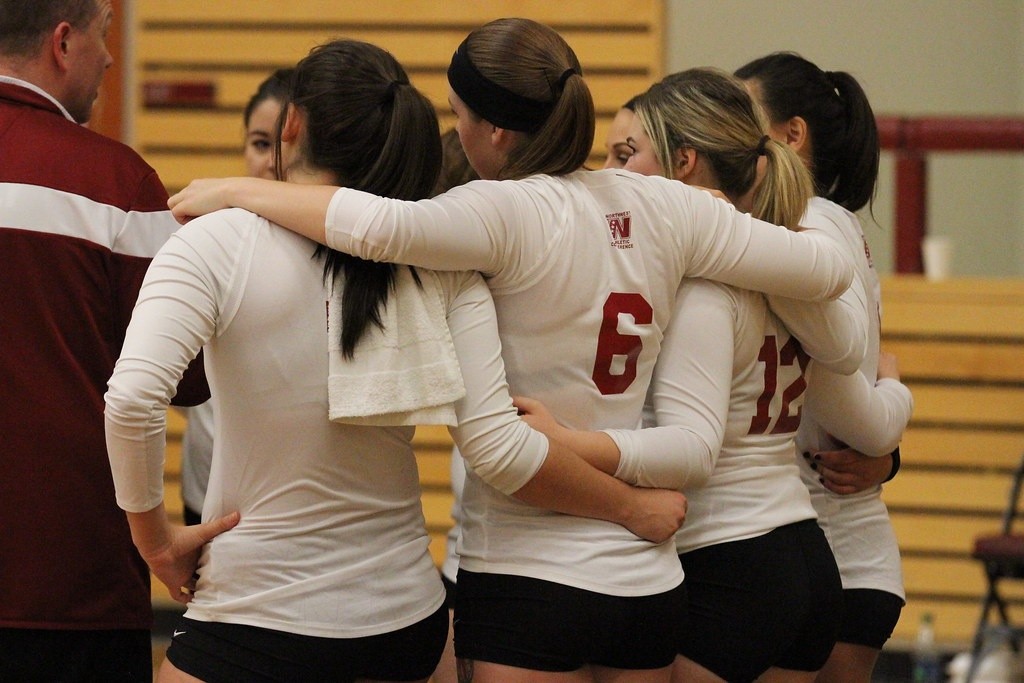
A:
912,612,939,683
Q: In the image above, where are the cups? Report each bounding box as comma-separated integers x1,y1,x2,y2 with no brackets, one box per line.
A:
921,237,954,278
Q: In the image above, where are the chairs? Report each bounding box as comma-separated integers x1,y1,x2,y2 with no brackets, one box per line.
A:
967,457,1024,683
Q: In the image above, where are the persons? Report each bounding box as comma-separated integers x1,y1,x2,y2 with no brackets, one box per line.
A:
513,53,911,683
0,0,210,683
165,16,852,683
101,38,687,683
